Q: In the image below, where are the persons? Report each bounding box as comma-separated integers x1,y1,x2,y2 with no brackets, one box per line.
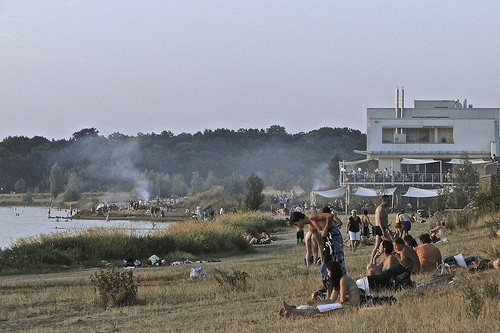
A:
414,234,443,272
282,262,360,317
393,237,420,274
369,195,392,265
97,153,500,244
289,211,347,293
365,241,400,275
304,232,319,266
